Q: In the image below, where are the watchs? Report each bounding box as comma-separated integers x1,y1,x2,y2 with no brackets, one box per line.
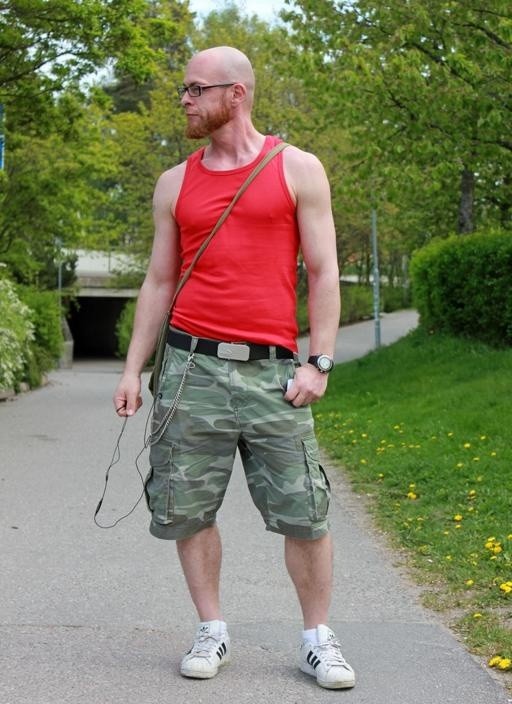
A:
306,355,336,374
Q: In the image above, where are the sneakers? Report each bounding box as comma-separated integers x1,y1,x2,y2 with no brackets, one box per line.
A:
300,624,355,689
180,620,230,678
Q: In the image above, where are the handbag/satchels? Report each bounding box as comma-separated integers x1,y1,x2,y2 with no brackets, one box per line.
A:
148,311,170,400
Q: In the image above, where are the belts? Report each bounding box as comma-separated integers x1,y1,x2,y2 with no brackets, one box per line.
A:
163,330,296,361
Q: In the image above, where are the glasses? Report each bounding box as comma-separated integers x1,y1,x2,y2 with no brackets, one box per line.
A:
177,84,235,97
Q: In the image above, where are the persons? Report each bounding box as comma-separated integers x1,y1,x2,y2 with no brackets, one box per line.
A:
110,44,367,692
